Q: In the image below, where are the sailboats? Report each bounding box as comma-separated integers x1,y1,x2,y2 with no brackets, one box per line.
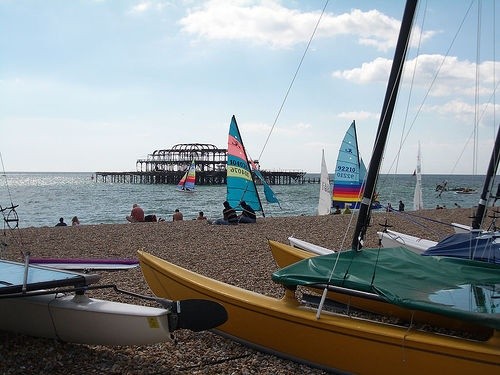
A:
135,0,499,374
175,158,195,195
332,119,382,212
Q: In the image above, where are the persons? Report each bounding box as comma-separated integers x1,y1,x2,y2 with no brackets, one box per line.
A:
126,204,144,222
332,204,341,214
55,218,67,226
72,216,80,225
173,209,183,221
399,201,404,212
386,203,394,212
197,211,207,220
239,200,256,223
342,205,351,214
214,201,238,225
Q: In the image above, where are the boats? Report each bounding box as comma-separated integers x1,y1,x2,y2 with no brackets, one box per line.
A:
376,222,499,261
1,251,229,349
